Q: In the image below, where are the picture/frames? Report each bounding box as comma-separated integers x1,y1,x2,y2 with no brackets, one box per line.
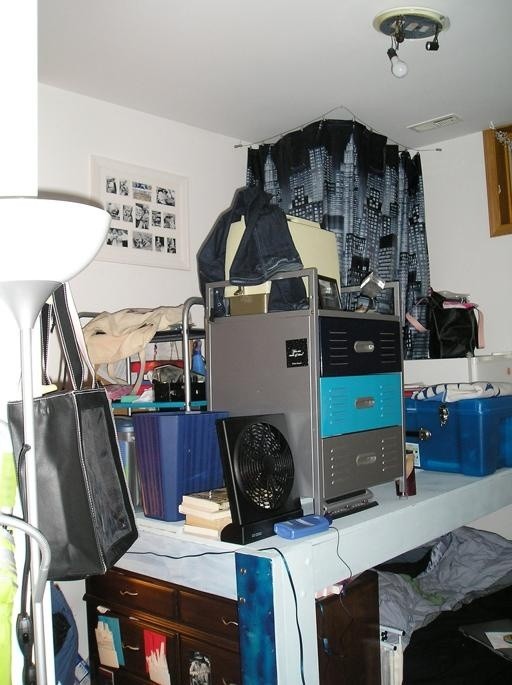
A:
90,155,190,272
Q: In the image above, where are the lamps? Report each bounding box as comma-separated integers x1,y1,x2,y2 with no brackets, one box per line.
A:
0,196,112,685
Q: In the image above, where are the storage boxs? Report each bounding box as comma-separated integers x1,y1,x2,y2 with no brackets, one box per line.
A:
403,399,511,478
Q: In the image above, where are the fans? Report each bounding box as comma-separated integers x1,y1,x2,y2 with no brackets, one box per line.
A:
214,410,304,547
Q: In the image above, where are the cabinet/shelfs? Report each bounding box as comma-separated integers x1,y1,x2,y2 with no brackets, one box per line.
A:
83,567,239,685
205,278,407,516
79,297,204,412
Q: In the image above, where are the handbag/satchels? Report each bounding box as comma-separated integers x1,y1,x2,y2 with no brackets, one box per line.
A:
425,289,480,359
83,305,190,365
6,387,138,583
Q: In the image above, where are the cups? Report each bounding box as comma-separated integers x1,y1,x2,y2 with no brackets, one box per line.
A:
395,449,416,496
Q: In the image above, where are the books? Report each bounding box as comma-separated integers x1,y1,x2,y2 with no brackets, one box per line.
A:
178,486,273,537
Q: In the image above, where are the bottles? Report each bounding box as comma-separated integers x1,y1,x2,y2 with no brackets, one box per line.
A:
115,416,142,513
192,349,207,378
